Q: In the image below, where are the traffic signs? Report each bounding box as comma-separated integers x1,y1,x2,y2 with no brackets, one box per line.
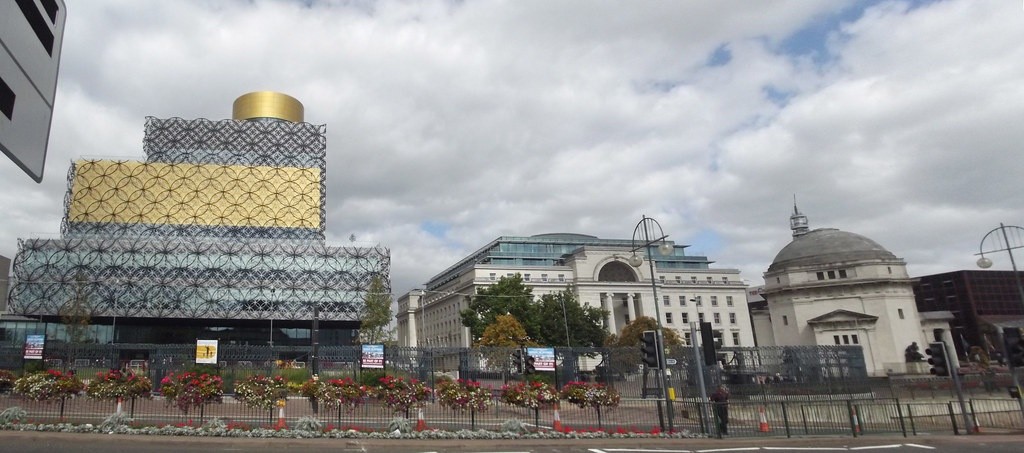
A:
665,358,677,365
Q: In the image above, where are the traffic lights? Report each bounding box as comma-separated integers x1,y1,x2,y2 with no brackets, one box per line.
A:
525,355,536,375
639,330,660,371
512,348,523,375
949,325,971,363
925,340,952,378
700,321,723,367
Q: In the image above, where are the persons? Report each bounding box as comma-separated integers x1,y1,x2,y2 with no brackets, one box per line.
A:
710,384,728,439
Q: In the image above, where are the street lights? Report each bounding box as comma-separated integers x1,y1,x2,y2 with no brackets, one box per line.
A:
972,222,1024,311
628,214,675,436
269,285,276,360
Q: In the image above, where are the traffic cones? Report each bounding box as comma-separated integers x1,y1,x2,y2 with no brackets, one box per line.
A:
116,397,123,414
851,406,860,432
278,406,287,430
755,405,770,433
416,407,426,433
552,403,563,433
971,413,982,432
